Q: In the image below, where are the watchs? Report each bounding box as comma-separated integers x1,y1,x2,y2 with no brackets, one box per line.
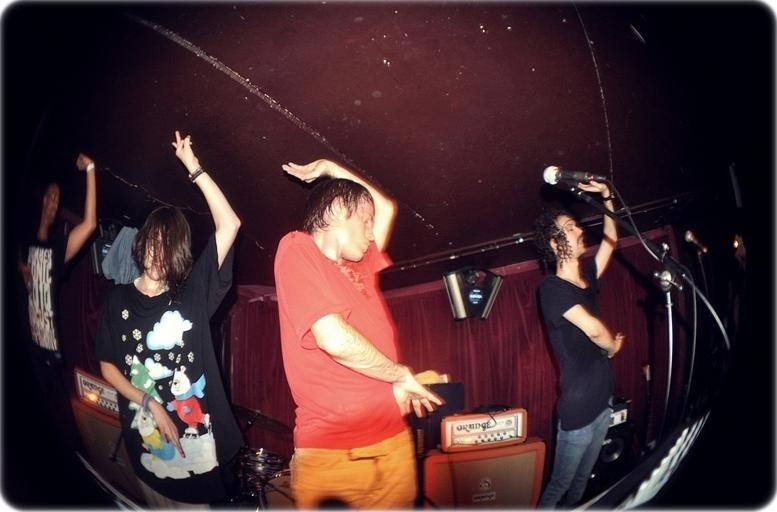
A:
187,165,207,184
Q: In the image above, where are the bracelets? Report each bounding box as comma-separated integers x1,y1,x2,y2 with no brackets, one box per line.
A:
85,161,96,172
141,392,154,412
601,194,612,202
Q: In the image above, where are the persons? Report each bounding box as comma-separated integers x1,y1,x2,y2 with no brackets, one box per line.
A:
16,149,99,433
531,176,629,508
272,158,442,508
99,128,249,509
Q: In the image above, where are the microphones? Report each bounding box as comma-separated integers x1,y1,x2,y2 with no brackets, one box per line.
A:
542,165,608,187
683,230,709,254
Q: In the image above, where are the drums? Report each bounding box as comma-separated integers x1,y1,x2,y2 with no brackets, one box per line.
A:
237,444,282,494
261,470,295,511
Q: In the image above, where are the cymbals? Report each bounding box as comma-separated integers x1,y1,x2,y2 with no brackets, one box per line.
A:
231,399,291,433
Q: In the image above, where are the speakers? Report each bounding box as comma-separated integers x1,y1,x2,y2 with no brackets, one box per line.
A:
590,418,643,491
